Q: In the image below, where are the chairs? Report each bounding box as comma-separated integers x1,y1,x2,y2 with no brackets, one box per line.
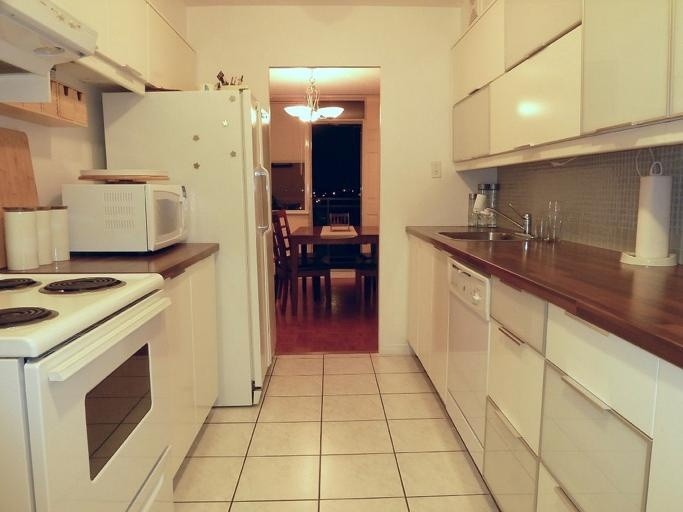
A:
271,208,379,315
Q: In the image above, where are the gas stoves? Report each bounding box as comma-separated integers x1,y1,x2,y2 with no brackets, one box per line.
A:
0,271,166,357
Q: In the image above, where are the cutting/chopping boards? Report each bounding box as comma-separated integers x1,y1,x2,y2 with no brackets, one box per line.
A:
0,127,40,273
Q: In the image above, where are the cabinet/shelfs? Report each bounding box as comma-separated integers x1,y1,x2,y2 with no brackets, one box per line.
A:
486,319,546,457
407,234,452,406
163,254,216,480
535,459,579,511
0,75,88,129
544,300,659,441
484,395,539,511
646,354,683,511
0,0,196,93
490,272,549,357
539,358,653,511
452,2,683,174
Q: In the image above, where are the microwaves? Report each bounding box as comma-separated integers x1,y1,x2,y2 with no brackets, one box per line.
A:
60,182,188,256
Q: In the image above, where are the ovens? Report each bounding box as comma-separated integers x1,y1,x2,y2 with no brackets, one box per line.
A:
22,299,187,512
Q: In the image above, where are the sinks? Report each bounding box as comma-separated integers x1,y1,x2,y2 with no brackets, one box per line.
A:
435,230,534,242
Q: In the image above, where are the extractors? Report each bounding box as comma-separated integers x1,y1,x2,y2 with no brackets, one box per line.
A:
0,0,99,105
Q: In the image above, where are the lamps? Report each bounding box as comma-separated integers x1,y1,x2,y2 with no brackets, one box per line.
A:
282,66,344,125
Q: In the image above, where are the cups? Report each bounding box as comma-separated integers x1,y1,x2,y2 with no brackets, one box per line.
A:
536,201,568,245
1,208,69,271
467,183,500,231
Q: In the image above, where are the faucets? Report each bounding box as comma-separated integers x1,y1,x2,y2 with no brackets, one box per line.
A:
484,202,532,234
